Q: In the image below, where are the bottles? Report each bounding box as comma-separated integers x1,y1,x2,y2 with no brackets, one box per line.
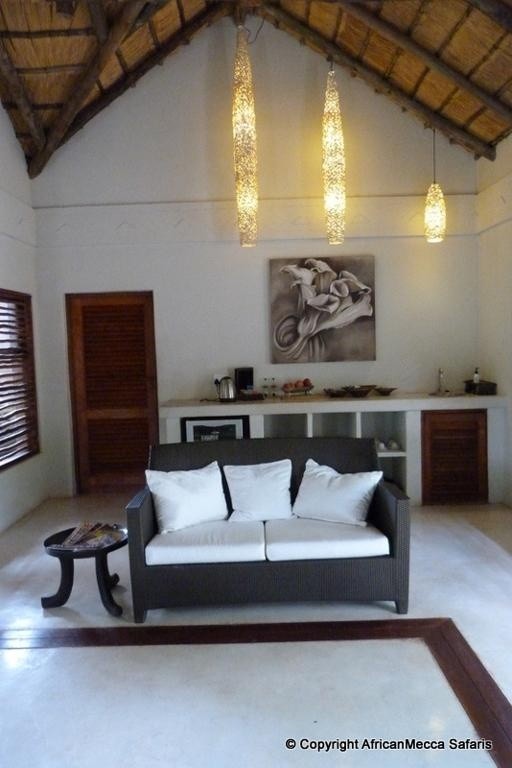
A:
263,377,277,400
472,367,480,384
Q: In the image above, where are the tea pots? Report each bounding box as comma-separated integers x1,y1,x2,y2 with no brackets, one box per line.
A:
215,377,238,402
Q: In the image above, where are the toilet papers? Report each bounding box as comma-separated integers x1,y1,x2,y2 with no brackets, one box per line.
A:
473,368,480,383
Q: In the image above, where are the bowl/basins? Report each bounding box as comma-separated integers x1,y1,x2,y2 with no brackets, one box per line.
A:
374,388,396,395
340,385,376,397
321,386,352,397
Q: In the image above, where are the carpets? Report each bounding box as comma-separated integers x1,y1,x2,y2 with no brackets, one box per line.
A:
0,491,512,768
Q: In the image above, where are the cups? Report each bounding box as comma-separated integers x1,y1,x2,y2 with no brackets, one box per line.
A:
376,439,399,451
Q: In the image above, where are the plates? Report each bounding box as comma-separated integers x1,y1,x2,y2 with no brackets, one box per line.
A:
281,385,314,393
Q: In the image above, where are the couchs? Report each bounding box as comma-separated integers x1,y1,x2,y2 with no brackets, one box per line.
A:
126,437,410,624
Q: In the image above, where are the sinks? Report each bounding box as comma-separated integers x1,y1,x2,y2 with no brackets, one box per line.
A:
429,393,472,397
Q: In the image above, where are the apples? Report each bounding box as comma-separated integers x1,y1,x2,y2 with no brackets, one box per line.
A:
284,379,311,388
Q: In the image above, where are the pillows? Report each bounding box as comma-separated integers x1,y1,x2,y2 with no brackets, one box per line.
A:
223,459,297,521
145,461,227,535
293,458,383,527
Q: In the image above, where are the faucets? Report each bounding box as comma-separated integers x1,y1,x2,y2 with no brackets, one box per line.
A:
439,368,445,393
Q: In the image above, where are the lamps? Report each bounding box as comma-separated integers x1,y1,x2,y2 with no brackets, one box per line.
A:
424,129,446,242
232,0,257,248
321,56,346,245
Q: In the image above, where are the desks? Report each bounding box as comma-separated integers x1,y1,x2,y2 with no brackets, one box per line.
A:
159,399,510,504
